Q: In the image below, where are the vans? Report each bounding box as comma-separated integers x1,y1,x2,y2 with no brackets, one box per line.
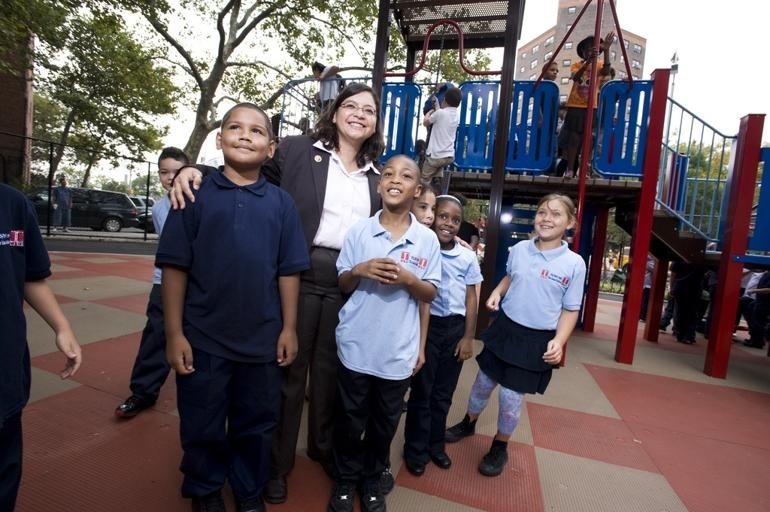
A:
34,185,157,231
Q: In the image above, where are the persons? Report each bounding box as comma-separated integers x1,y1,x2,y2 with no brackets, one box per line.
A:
457,221,479,250
536,58,565,137
114,145,190,419
401,189,485,476
603,254,611,270
1,182,84,512
51,174,72,235
151,102,312,511
298,117,313,136
558,99,568,121
166,82,385,507
561,30,617,179
611,255,620,270
638,256,769,350
444,190,588,477
419,89,463,186
598,68,616,88
307,60,340,114
378,185,436,497
325,150,443,512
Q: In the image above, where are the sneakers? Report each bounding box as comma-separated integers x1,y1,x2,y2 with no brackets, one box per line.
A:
329,480,357,512
562,167,575,179
378,464,395,495
479,435,512,477
358,475,387,511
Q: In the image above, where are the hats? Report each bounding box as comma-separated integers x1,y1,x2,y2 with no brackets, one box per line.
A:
576,35,605,59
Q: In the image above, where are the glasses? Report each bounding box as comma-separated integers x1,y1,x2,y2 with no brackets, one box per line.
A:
339,100,377,115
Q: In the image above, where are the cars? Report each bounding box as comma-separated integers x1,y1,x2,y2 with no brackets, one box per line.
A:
614,246,631,273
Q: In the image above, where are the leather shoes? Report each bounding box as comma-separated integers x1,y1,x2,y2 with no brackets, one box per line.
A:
231,488,266,512
432,449,452,470
444,412,478,443
655,320,770,350
406,461,426,477
264,475,289,504
305,448,342,480
191,491,227,511
115,392,157,420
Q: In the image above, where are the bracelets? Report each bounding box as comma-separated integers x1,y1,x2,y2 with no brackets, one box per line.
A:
583,60,591,66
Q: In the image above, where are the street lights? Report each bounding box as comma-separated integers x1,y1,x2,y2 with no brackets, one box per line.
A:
669,63,678,100
